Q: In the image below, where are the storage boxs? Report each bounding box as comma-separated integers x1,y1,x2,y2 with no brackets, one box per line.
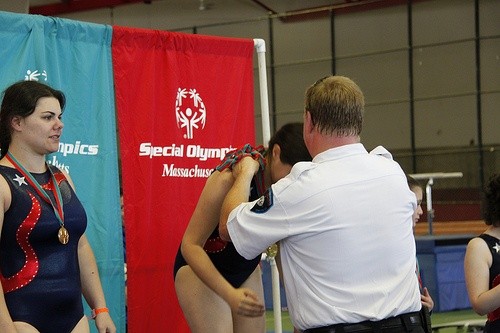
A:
415,236,473,312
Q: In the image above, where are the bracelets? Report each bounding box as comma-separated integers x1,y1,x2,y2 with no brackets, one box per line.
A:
90,307,110,319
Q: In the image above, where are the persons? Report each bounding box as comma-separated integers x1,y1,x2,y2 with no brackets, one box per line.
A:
402,173,434,333
463,169,500,333
171,122,315,333
0,81,119,333
219,73,429,333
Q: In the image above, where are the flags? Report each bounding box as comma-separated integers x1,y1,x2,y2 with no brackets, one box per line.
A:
0,10,126,332
108,24,256,333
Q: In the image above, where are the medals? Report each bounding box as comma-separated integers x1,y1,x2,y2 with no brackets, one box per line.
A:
5,147,69,244
251,156,280,258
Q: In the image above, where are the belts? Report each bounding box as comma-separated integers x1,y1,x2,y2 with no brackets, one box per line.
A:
330,312,421,332
